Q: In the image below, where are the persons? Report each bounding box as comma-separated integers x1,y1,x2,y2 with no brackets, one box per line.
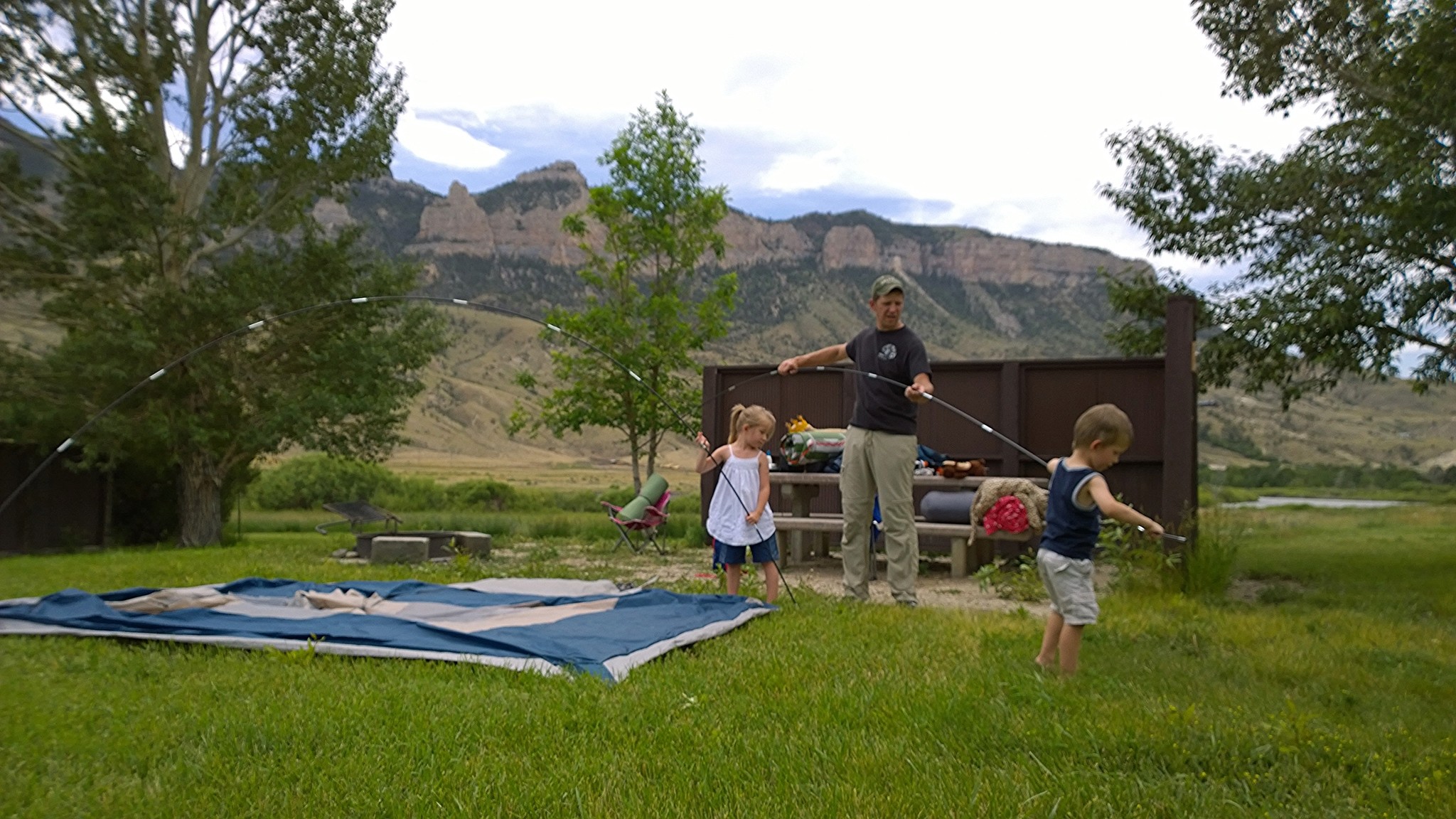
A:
776,275,936,606
694,404,778,606
1034,403,1163,676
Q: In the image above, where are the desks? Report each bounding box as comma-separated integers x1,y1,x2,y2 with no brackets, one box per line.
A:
769,473,1050,579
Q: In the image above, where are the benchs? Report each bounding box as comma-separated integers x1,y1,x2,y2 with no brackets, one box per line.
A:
774,512,1034,579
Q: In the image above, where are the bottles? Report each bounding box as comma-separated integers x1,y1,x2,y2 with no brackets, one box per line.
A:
765,451,772,469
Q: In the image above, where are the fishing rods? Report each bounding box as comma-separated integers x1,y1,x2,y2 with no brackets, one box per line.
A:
629,367,1187,543
1,295,798,606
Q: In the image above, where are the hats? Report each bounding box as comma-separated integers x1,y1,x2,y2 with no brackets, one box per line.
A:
872,274,905,299
